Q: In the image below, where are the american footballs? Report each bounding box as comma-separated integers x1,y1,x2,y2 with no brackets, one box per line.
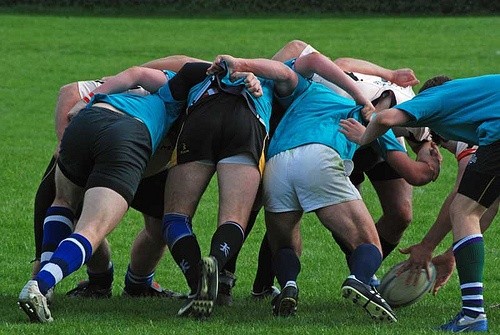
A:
379,259,436,307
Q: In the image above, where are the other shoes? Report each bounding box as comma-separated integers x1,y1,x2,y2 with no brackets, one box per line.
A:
370,273,381,286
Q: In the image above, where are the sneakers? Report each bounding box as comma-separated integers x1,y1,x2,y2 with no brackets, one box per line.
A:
340,275,398,323
179,256,218,318
122,274,187,299
18,280,54,323
272,280,299,316
66,281,113,298
440,312,487,332
218,269,239,306
251,287,282,298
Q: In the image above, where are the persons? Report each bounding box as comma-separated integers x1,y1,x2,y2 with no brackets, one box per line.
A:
338,74,500,334
19,38,442,326
393,127,500,297
